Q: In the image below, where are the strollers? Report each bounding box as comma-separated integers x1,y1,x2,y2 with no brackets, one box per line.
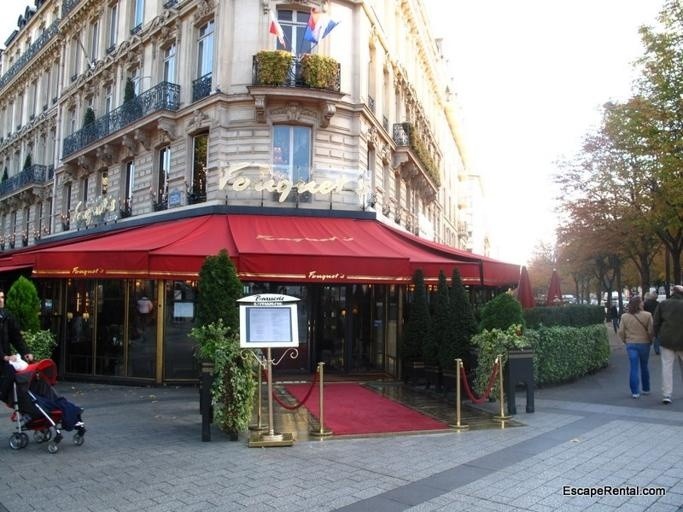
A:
7,355,87,455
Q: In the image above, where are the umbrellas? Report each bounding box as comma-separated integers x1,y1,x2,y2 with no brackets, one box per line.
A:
546,269,564,307
518,266,536,310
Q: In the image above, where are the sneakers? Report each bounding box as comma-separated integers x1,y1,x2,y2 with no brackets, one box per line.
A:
640,389,649,394
633,393,640,399
662,396,672,406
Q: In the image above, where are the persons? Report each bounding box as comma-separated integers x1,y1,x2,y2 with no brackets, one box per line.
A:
654,285,683,402
0,289,33,408
644,292,660,355
611,304,621,333
618,297,655,398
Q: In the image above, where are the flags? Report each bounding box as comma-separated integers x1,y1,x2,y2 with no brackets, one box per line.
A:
269,11,286,49
319,16,336,38
304,13,321,44
